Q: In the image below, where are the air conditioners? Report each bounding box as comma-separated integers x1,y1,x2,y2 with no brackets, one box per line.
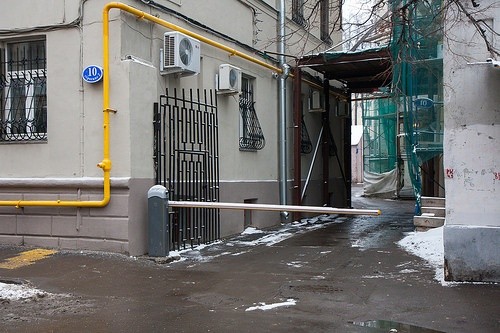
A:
337,101,349,116
218,63,242,92
163,30,201,74
312,90,326,110
